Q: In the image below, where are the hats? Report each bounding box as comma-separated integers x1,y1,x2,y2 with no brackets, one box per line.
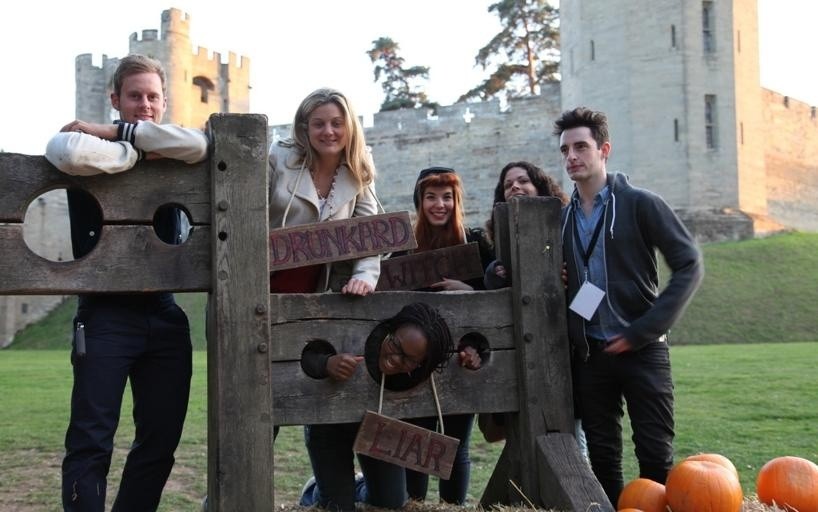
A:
413,167,454,207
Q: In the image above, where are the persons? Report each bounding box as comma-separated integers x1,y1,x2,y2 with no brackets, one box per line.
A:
387,168,485,504
553,108,705,506
266,88,381,442
477,161,569,440
46,53,206,512
302,303,456,512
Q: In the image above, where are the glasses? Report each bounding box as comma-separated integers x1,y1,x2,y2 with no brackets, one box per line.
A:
387,333,418,373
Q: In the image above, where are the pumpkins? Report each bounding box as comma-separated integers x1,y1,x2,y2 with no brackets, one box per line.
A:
615,454,818,511
